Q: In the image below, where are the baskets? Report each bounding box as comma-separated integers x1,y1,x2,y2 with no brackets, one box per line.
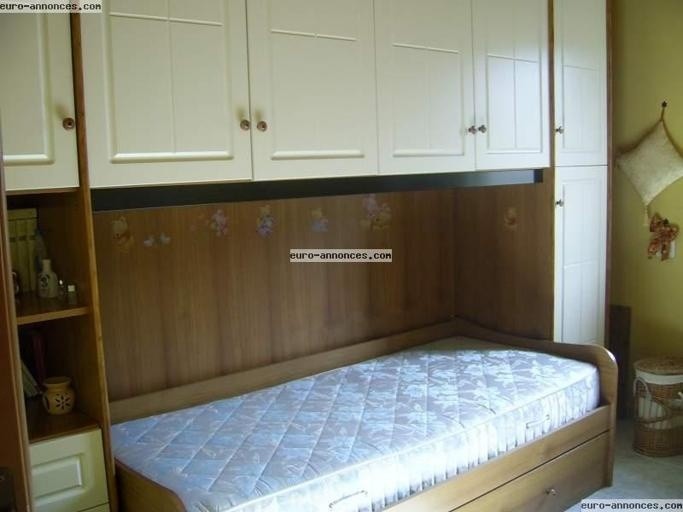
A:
632,376,683,456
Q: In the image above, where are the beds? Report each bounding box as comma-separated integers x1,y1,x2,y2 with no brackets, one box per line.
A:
104,322,619,512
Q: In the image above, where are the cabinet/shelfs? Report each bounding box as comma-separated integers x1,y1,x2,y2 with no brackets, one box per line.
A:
551,1,615,166
373,1,548,178
0,192,118,510
76,0,379,188
0,2,80,199
453,169,610,347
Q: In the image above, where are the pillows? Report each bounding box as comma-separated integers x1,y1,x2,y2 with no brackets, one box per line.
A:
613,121,682,225
